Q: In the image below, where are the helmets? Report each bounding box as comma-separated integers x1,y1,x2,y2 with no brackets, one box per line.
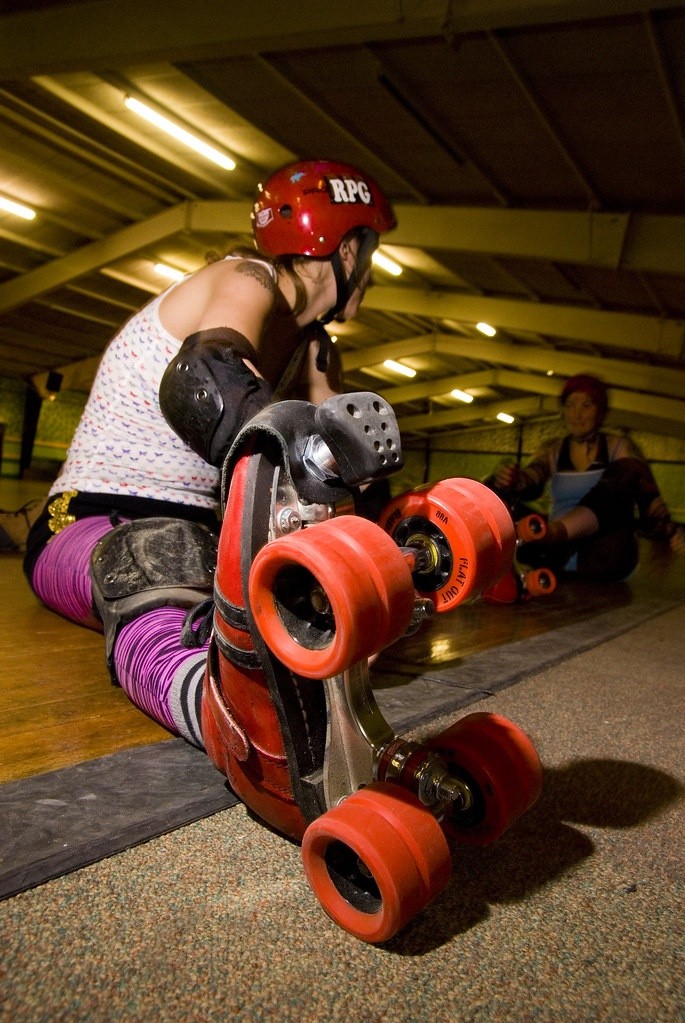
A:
559,373,609,419
249,158,398,259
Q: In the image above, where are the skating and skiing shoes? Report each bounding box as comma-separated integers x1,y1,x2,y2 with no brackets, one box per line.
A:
511,513,557,606
177,391,542,943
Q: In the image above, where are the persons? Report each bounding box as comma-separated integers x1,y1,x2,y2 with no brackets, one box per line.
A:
24,158,542,944
496,374,685,595
